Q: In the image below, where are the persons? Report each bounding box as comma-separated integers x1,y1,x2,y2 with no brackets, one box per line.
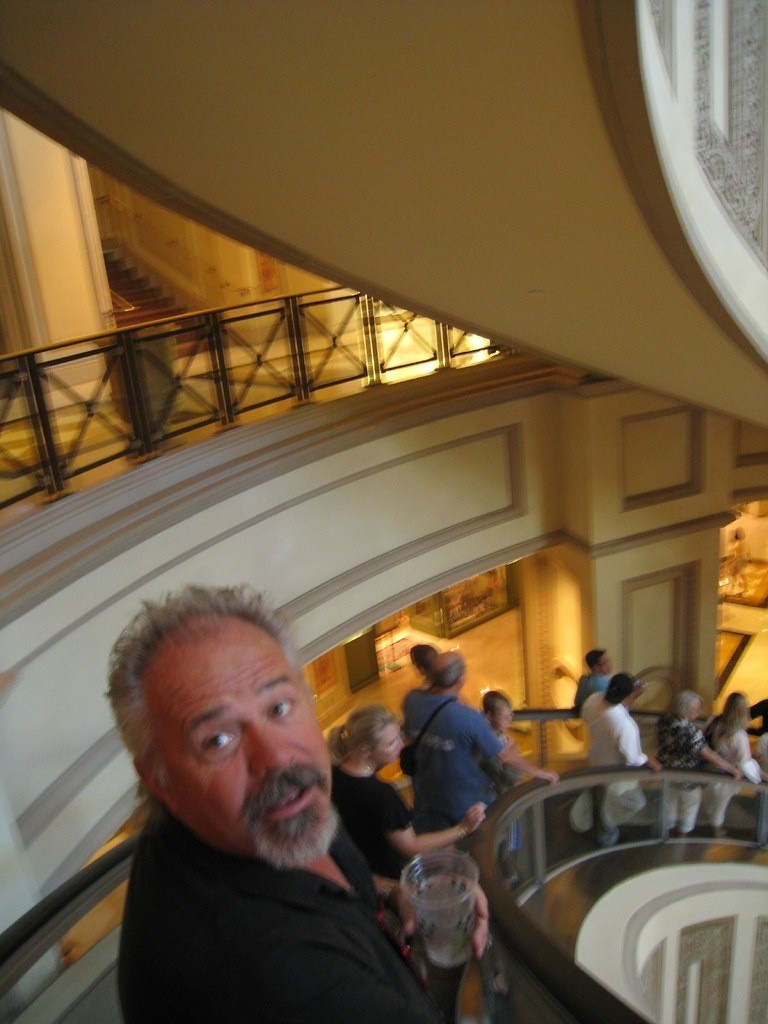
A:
580,671,663,846
102,578,490,1024
400,645,558,890
701,692,768,833
330,708,487,954
655,690,740,835
746,697,768,845
569,649,648,717
482,689,524,881
410,644,469,706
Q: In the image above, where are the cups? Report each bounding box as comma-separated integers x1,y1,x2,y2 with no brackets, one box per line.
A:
401,847,480,968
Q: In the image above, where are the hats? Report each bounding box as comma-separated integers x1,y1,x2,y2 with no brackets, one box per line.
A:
583,649,606,667
602,672,634,704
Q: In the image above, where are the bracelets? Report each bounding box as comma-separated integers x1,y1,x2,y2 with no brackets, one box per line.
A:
457,824,467,838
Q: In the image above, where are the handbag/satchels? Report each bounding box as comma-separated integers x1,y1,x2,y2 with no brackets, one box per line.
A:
397,698,459,777
